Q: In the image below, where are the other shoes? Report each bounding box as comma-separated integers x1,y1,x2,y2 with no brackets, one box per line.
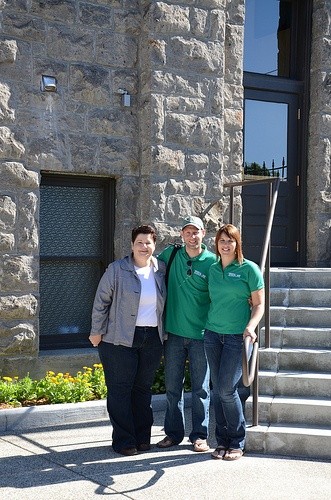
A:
192,437,210,452
211,446,227,459
120,448,138,456
156,436,178,448
223,448,244,461
137,444,150,451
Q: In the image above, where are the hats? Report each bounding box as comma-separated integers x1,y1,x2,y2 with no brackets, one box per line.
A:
182,215,204,230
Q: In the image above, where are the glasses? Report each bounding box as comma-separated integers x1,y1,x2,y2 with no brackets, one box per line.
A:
187,260,192,276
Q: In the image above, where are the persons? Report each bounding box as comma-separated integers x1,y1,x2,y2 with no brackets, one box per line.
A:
155,215,253,453
203,224,266,461
89,224,167,456
